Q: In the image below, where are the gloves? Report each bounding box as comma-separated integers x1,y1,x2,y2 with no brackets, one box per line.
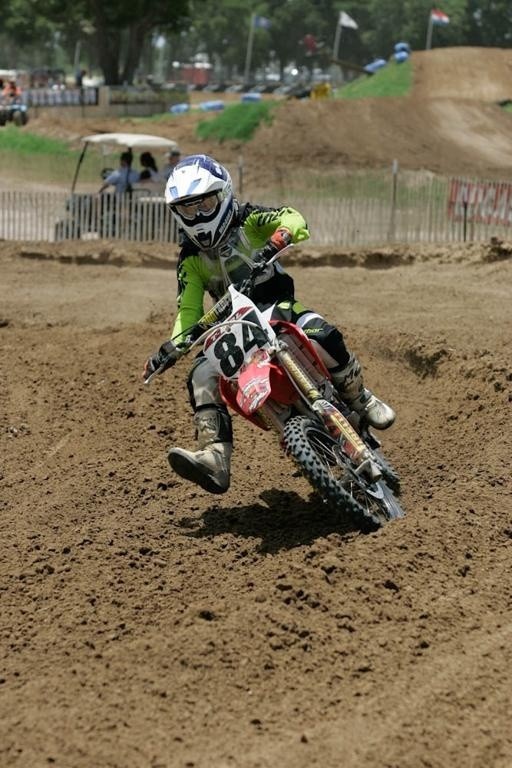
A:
142,353,161,378
266,227,292,249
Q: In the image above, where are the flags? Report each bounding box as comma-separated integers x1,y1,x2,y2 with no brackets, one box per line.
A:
255,15,272,29
341,11,358,30
431,8,448,26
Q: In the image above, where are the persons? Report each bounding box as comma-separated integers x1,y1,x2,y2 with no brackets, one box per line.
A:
51,77,68,90
137,151,159,182
77,70,85,85
0,79,21,106
100,147,139,195
142,154,396,495
163,151,181,177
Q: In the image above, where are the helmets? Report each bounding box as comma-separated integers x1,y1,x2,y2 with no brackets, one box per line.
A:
164,154,234,251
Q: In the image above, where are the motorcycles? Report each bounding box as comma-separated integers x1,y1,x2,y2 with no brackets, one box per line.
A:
142,232,404,534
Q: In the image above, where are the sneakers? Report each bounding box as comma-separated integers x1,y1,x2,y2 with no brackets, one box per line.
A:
348,388,396,430
167,441,233,494
